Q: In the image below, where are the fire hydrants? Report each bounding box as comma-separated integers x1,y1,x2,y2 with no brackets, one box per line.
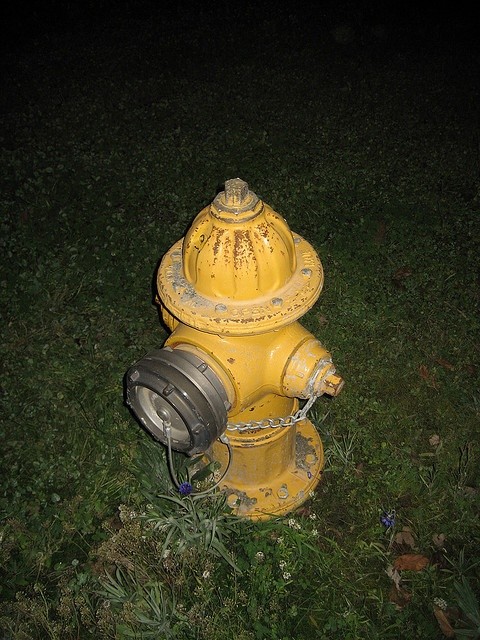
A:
124,178,345,523
